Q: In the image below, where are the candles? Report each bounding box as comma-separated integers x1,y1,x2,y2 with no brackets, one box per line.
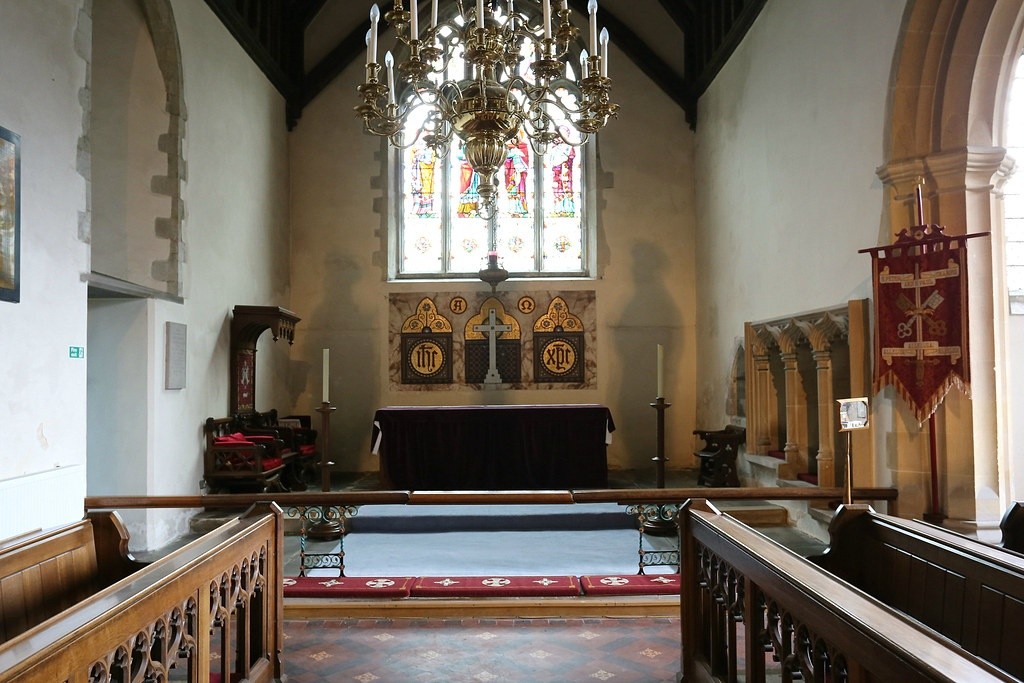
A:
323,347,330,403
656,344,664,397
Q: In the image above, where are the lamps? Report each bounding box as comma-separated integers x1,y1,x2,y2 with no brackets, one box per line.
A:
353,0,618,220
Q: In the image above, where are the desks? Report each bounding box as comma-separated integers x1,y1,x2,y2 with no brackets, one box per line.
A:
370,407,616,489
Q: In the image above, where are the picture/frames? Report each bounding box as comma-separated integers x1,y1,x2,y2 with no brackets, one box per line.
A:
1,126,21,303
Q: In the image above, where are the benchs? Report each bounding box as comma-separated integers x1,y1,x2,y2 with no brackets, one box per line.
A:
0,510,154,648
798,505,1024,683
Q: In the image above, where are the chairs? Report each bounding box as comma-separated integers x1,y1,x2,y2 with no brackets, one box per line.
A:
693,425,745,486
204,407,319,497
